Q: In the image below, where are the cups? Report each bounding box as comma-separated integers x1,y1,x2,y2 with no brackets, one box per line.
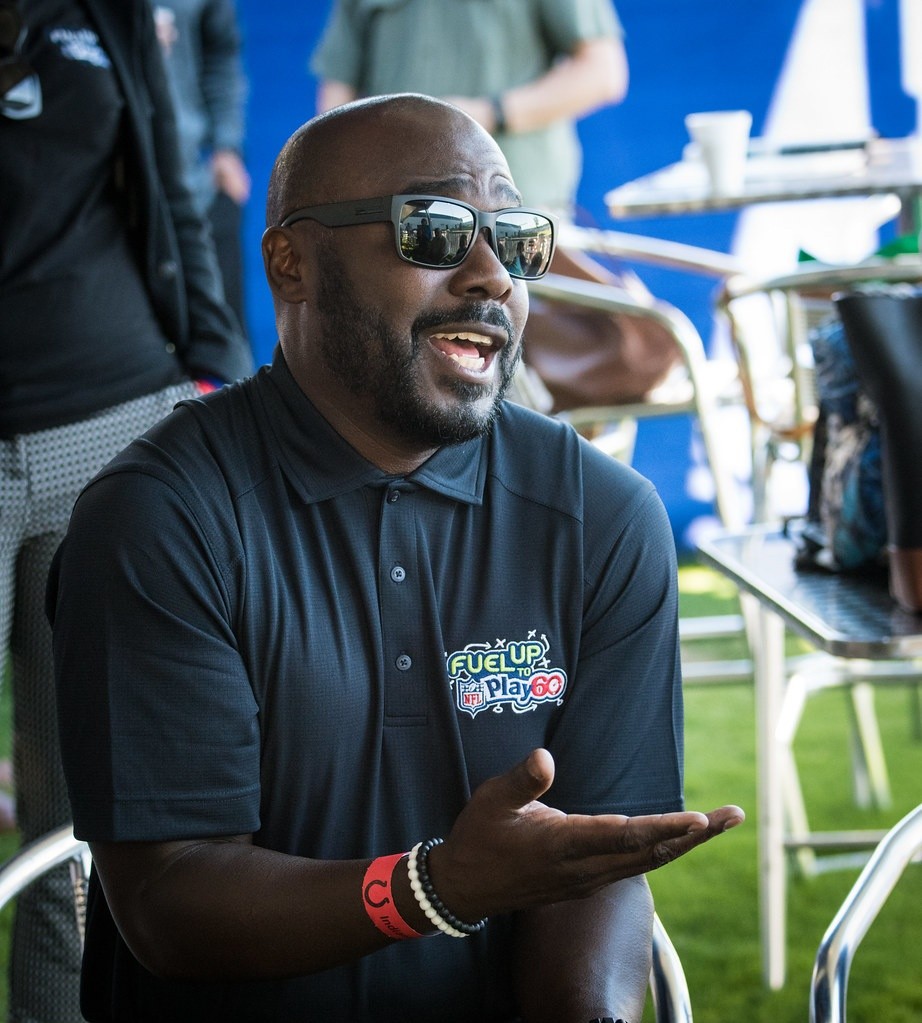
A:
684,110,753,198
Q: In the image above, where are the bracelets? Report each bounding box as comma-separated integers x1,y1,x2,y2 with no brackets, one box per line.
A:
492,97,506,133
361,852,443,941
408,838,487,938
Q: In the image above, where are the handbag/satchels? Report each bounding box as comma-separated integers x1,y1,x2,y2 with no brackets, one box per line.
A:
795,286,922,617
521,237,681,415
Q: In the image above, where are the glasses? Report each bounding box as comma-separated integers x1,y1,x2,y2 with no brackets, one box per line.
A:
279,194,557,280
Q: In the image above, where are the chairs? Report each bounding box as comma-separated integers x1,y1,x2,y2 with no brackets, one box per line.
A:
525,222,922,881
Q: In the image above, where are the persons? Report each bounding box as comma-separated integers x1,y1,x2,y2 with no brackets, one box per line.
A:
314,0,629,223
0,0,267,1023
45,93,746,1023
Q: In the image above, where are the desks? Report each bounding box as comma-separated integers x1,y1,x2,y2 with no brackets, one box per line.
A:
605,137,922,1023
